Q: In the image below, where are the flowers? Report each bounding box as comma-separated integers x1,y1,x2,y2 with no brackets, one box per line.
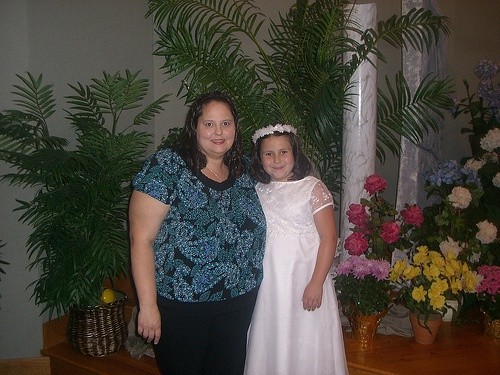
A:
331,58,500,335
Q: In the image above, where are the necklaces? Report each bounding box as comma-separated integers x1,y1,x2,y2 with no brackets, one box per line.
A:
206,164,225,181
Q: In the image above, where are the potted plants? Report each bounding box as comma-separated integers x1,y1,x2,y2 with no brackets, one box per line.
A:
0,65,176,358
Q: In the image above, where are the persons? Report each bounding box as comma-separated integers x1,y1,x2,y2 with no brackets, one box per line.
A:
244,123,349,375
130,90,267,375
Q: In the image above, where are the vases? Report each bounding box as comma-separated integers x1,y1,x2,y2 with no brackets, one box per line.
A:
409,310,443,345
354,315,378,345
483,314,500,339
436,298,464,322
